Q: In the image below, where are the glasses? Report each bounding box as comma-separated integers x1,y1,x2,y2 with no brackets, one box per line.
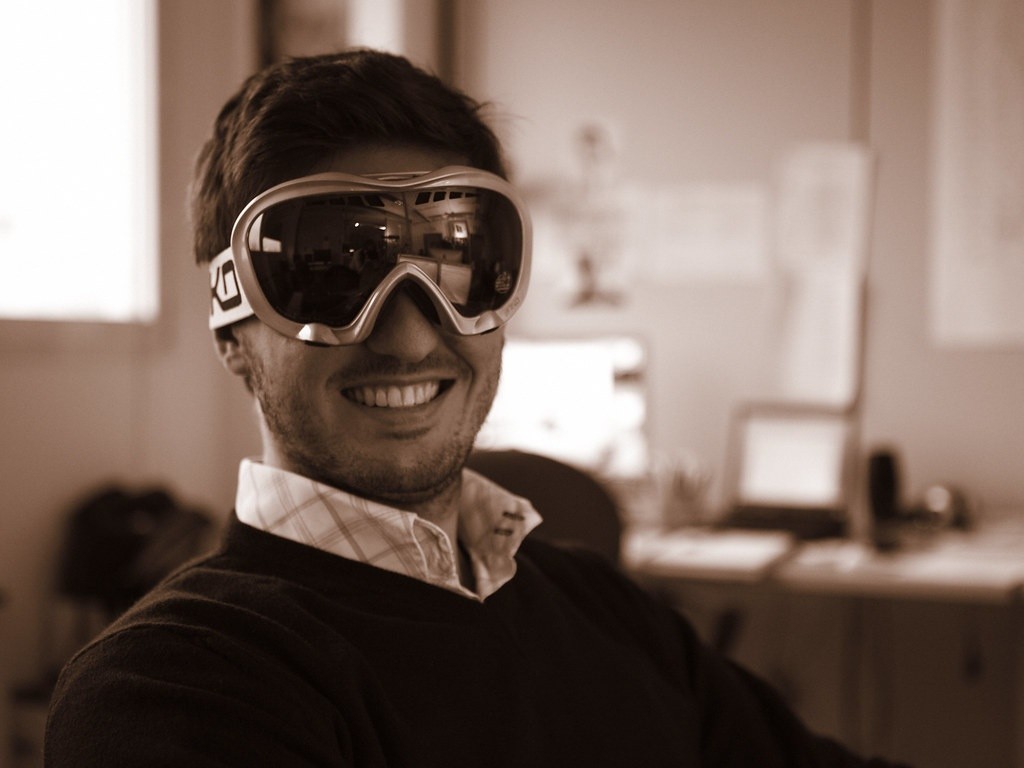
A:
207,164,535,346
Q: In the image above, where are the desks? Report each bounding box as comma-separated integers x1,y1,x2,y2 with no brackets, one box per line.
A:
623,505,1023,767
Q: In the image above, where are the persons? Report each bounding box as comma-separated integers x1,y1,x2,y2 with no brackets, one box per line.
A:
43,51,894,768
